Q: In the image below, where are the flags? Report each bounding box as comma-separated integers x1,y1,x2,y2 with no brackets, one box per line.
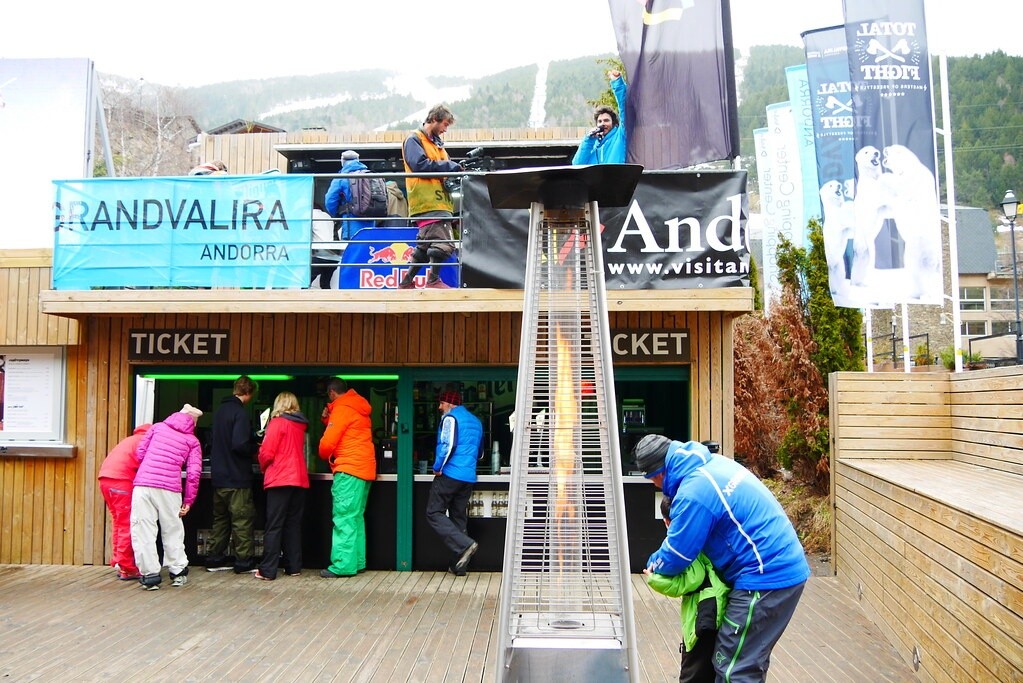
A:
608,0,731,172
753,0,945,317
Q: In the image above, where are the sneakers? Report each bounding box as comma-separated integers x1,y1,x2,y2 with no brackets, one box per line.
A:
321,570,357,578
171,575,187,586
254,571,274,580
358,568,366,573
425,278,450,288
284,569,301,576
398,282,418,289
234,563,258,574
138,574,162,590
206,558,234,572
455,542,478,569
448,567,466,576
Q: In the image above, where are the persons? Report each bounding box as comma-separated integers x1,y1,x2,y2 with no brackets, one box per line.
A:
130,403,203,590
396,105,465,289
572,71,627,166
300,149,408,288
97,424,153,581
426,390,485,576
205,376,310,581
635,434,811,683
189,160,228,290
319,378,377,578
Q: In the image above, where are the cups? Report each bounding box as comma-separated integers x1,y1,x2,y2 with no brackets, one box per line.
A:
492,441,500,475
419,461,428,474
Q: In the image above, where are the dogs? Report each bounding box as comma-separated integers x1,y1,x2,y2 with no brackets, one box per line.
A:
189,161,228,175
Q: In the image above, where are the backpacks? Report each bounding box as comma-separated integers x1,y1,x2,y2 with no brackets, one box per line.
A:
336,170,389,219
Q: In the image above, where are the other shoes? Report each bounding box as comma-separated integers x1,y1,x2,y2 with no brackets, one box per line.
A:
117,571,141,582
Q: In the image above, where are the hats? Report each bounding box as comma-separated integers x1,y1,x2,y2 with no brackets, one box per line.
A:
438,388,461,406
341,151,359,167
180,404,203,427
636,434,672,479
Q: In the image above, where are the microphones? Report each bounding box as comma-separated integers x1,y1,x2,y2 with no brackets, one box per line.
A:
466,148,484,158
590,127,605,136
